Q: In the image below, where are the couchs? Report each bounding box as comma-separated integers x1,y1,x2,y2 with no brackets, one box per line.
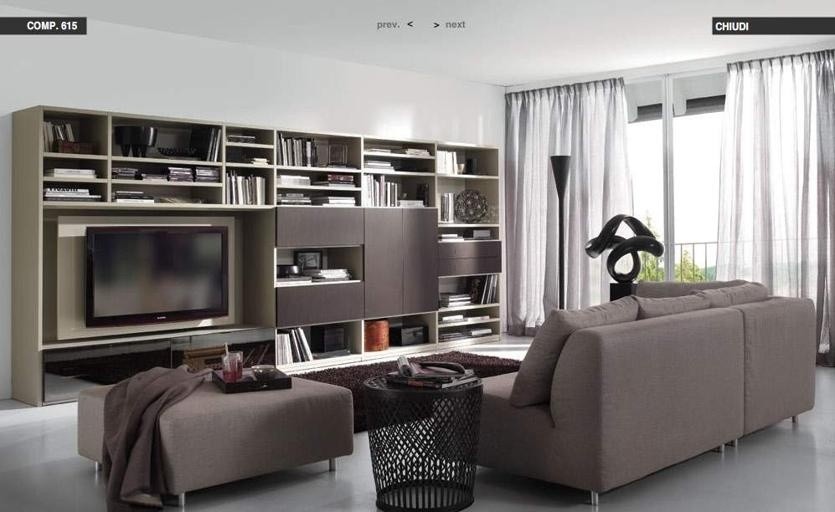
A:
437,280,818,506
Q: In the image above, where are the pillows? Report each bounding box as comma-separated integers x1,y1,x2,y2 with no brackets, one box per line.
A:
509,284,765,409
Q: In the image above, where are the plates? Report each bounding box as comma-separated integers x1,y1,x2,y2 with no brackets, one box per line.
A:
454,188,490,223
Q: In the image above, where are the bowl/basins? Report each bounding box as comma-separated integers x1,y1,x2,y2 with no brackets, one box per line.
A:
277,265,301,278
251,364,275,371
253,367,277,380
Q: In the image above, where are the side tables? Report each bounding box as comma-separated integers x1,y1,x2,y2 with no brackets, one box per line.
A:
366,371,481,511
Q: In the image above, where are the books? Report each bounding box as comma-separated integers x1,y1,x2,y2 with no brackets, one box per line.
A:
275,232,501,368
242,340,272,370
385,375,482,389
39,117,459,224
385,367,475,383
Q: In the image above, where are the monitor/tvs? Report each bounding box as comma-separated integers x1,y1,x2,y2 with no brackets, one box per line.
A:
84,225,228,326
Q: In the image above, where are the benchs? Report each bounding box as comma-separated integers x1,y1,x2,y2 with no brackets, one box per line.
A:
77,372,352,512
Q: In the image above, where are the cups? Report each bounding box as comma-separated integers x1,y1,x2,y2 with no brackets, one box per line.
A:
229,351,243,379
457,163,465,174
443,191,456,222
465,157,478,175
222,354,238,383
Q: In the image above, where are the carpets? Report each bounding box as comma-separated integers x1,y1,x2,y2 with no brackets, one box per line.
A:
289,351,525,433
